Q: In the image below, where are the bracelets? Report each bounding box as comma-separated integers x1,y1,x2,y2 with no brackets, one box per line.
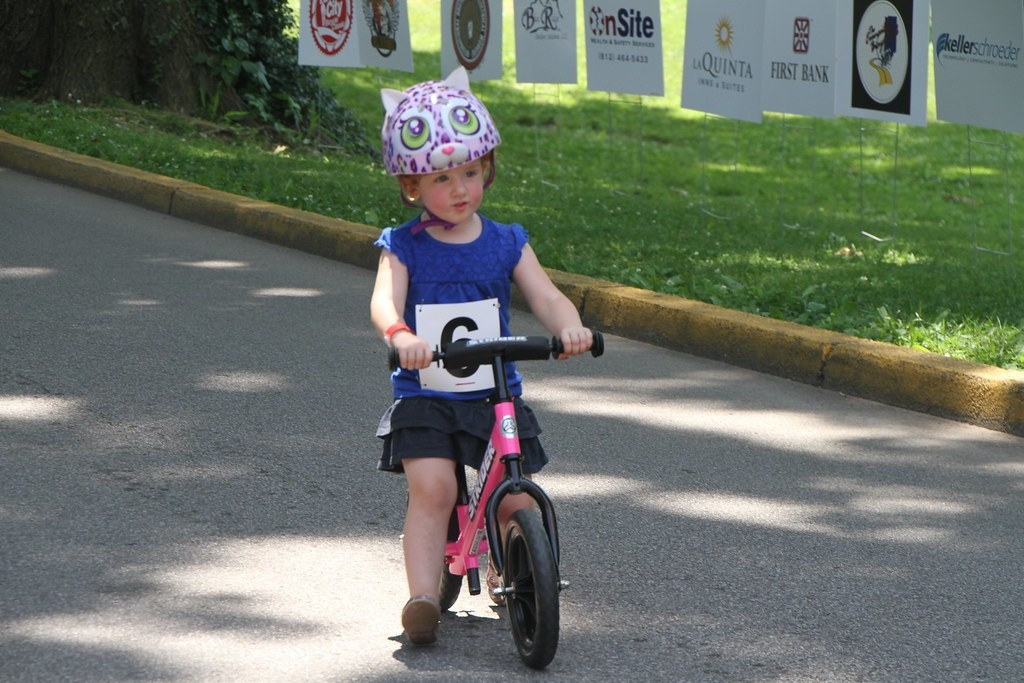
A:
384,322,413,347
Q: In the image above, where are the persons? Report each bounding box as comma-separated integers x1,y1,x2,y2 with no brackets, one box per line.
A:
370,66,593,645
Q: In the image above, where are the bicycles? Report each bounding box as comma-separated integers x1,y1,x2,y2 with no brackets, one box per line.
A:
388,329,603,670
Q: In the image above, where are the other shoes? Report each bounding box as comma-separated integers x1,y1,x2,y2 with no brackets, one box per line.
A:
486,550,506,605
401,594,439,645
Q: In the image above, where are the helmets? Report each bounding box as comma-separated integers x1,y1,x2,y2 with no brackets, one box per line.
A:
379,66,501,176
